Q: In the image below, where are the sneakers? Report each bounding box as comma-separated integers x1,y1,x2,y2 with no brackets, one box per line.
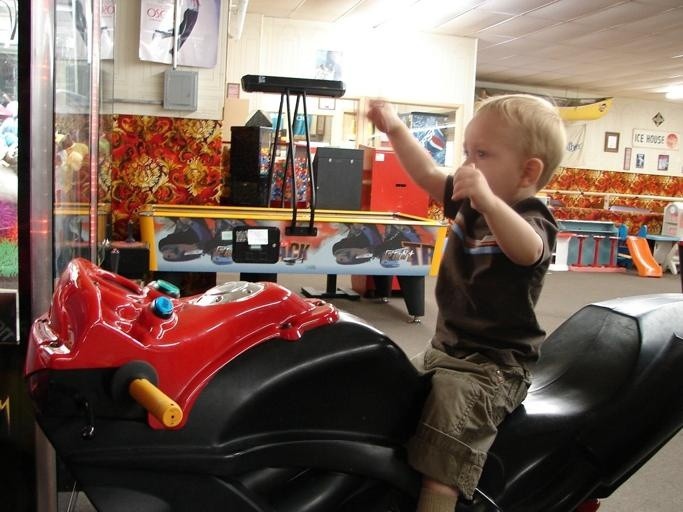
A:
333,222,375,255
159,223,206,260
377,225,421,258
209,219,248,257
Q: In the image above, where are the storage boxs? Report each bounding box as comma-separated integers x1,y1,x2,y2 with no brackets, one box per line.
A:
313,147,364,211
360,144,430,218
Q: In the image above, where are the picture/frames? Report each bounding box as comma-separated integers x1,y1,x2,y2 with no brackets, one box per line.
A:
604,132,620,152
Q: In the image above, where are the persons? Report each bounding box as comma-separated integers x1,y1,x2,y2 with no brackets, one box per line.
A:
152,1,202,54
367,93,566,512
